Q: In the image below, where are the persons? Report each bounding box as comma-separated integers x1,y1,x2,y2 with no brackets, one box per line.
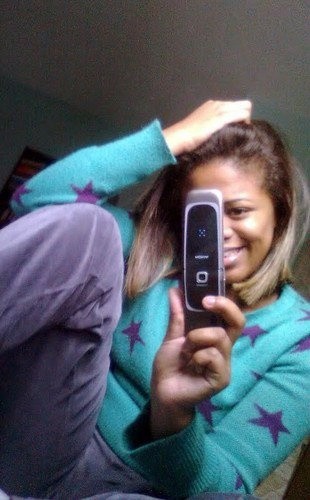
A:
0,100,310,500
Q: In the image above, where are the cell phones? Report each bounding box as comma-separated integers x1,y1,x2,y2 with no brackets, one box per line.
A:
183,189,225,314
